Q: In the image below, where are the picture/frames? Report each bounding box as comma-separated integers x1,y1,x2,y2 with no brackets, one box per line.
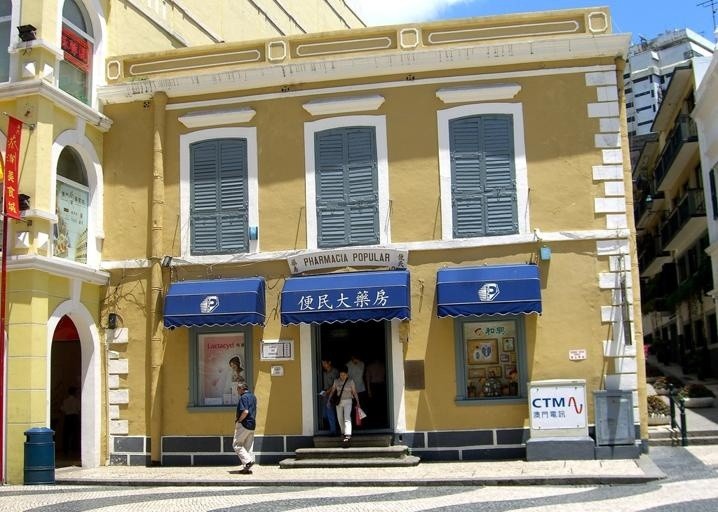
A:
467,337,516,379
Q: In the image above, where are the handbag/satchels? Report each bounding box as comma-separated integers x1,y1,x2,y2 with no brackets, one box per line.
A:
330,389,340,406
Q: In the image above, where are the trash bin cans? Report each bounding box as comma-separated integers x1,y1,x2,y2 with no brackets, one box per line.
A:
24,427,56,485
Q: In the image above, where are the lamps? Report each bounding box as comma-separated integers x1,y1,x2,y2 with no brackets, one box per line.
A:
19,194,30,210
161,256,172,269
17,24,36,42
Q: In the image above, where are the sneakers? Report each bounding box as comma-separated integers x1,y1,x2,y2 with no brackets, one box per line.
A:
243,462,255,471
341,434,351,444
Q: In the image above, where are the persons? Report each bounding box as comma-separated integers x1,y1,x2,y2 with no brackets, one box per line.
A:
232,381,257,474
364,353,387,429
326,363,360,444
229,355,245,382
318,354,340,437
345,352,367,429
59,385,81,461
